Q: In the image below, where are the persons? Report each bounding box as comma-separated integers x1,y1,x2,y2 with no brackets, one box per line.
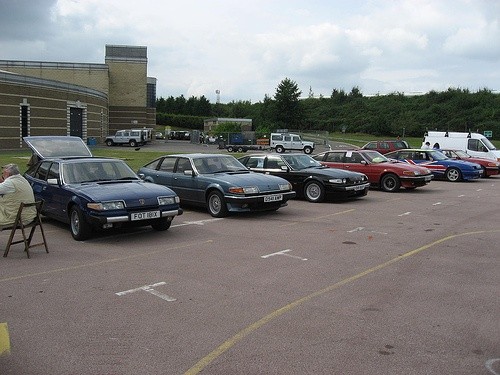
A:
0,165,37,226
421,142,431,149
432,143,442,153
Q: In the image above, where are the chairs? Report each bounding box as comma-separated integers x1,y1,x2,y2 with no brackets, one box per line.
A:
0,199,48,258
208,165,217,171
183,161,192,170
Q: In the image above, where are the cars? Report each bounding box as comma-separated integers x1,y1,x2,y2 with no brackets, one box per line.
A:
137,153,296,218
435,148,500,179
311,149,434,193
155,130,190,141
383,149,484,183
21,135,183,241
224,152,371,203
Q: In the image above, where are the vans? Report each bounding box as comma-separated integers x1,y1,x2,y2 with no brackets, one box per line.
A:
421,131,500,162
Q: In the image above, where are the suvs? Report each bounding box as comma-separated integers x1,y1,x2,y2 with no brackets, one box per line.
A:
270,133,315,155
129,127,152,144
104,129,144,147
360,139,409,155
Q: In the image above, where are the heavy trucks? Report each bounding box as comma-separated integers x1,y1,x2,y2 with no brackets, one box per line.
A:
224,133,270,154
